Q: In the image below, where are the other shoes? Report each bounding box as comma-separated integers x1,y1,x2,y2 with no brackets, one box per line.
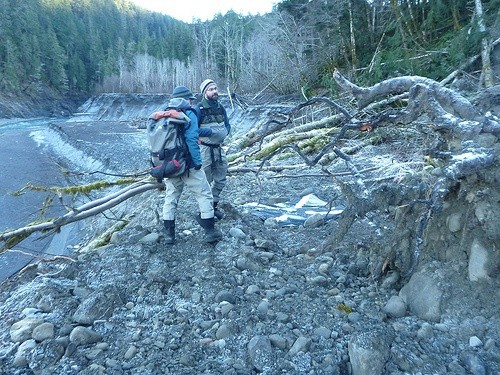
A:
195,215,204,228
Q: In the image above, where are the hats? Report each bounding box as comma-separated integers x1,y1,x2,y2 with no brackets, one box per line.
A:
173,85,199,100
200,79,215,95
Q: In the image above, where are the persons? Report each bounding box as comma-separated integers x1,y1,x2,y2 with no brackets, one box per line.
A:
155,85,223,245
194,78,231,221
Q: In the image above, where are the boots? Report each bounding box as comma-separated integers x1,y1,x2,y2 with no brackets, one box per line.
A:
163,220,176,243
212,203,224,219
201,214,220,242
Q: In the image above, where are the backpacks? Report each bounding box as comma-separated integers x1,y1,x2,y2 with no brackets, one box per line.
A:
146,107,198,183
